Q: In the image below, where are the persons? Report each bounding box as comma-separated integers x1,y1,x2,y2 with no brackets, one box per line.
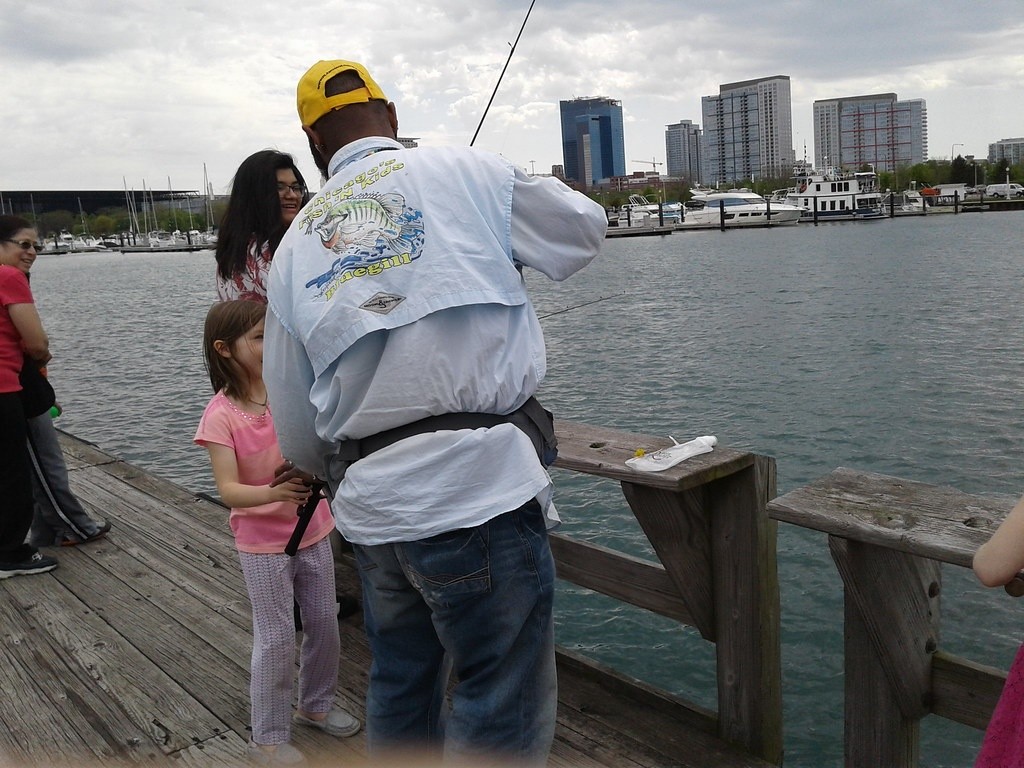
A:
0,214,111,578
972,493,1024,768
214,148,311,307
262,59,607,768
191,297,361,768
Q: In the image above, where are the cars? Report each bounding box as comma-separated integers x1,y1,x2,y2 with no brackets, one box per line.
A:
965,184,988,194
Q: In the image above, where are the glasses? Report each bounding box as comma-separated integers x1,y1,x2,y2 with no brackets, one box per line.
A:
4,239,42,251
276,185,305,197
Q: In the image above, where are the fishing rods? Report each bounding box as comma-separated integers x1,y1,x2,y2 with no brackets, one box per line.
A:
283,0,537,559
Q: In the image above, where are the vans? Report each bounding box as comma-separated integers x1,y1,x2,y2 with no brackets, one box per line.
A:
986,183,1024,198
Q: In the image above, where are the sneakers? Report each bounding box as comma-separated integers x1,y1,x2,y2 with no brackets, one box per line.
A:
0,543,58,579
293,709,360,737
246,735,308,768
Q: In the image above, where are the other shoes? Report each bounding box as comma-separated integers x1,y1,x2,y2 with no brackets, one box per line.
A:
293,594,357,632
30,535,55,547
55,521,111,546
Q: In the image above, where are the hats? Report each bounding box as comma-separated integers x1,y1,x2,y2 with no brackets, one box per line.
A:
297,60,387,127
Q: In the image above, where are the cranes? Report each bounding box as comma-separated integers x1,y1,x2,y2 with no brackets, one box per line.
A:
632,157,663,172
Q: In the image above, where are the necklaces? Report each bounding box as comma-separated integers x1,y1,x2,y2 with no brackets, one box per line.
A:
247,392,267,406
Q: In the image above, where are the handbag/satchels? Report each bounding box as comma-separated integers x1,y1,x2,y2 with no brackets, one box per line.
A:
18,352,55,418
323,396,558,500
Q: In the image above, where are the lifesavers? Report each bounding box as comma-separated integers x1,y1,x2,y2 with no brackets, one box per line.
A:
800,184,807,193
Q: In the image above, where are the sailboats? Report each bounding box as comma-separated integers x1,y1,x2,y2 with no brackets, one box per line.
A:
30,161,218,255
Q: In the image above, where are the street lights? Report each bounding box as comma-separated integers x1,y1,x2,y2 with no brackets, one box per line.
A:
951,143,963,161
529,160,536,176
971,164,982,187
874,153,887,172
1005,167,1011,200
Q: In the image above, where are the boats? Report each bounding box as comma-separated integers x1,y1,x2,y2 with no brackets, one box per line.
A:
607,140,962,237
